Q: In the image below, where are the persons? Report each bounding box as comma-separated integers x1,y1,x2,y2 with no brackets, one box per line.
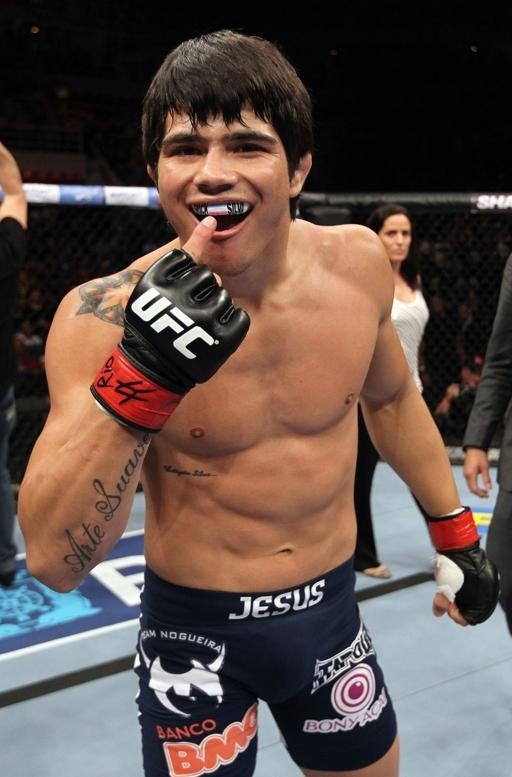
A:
356,576,360,578
16,29,497,777
0,141,30,588
462,242,510,642
363,201,432,580
